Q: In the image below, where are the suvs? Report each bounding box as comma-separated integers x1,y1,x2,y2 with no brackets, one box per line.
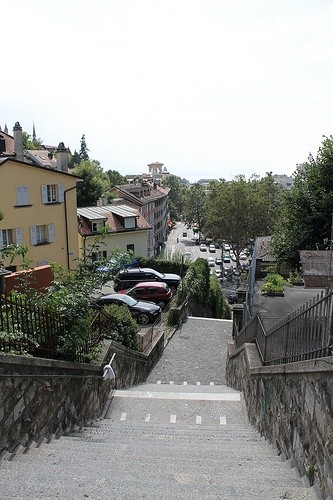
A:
113,267,182,294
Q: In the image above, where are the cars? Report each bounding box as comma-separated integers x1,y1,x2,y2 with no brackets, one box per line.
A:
115,280,172,309
191,221,252,274
88,292,162,325
